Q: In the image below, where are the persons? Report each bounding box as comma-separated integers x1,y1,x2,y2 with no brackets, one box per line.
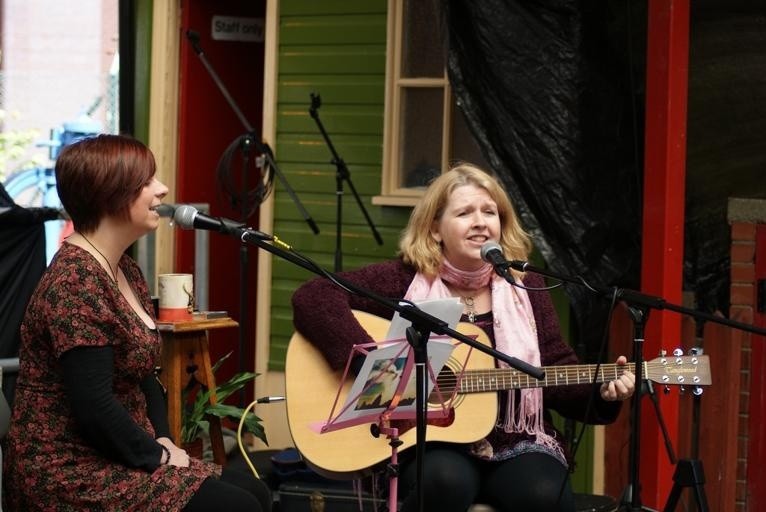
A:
0,184,71,415
0,133,271,512
290,159,636,512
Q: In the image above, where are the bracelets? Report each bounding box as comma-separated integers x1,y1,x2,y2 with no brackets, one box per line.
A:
163,445,171,466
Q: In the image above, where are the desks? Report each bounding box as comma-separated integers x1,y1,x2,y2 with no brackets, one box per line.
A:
157,317,240,469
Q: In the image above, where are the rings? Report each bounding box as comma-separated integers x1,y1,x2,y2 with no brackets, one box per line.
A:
622,395,629,403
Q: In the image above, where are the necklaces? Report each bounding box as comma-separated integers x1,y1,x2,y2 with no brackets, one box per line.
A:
76,230,118,290
450,285,490,325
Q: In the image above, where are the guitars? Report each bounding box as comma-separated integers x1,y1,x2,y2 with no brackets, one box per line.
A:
285,309,711,474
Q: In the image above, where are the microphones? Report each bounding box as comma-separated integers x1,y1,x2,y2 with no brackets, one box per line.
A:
480,238,518,286
173,204,278,243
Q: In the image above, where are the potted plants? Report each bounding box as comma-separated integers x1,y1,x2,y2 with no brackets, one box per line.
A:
172,350,269,461
